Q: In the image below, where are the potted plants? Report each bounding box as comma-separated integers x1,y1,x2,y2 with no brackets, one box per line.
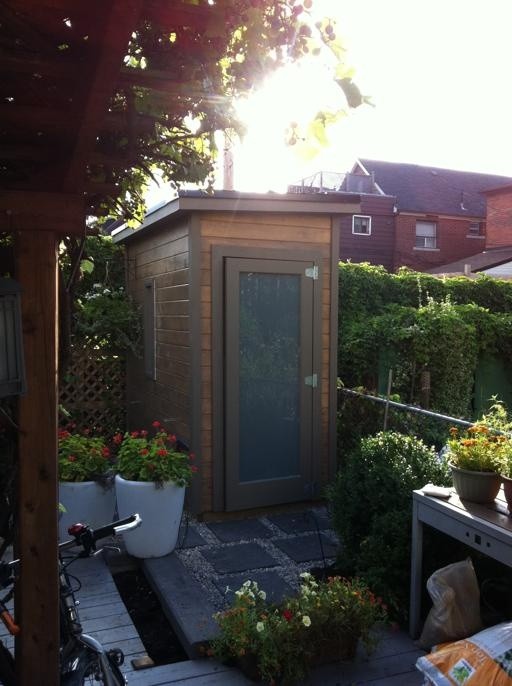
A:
500,439,512,514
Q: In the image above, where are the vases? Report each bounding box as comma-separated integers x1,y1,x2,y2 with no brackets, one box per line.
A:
303,629,358,669
115,472,186,559
448,459,501,504
57,481,116,554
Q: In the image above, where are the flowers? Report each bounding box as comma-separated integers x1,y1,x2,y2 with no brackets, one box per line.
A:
57,425,115,491
113,421,198,487
213,569,400,686
448,426,507,471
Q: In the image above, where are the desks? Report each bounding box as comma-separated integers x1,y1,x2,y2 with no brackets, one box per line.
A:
408,482,512,641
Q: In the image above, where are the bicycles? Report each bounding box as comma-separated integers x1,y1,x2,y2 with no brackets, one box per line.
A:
1,513,142,686
